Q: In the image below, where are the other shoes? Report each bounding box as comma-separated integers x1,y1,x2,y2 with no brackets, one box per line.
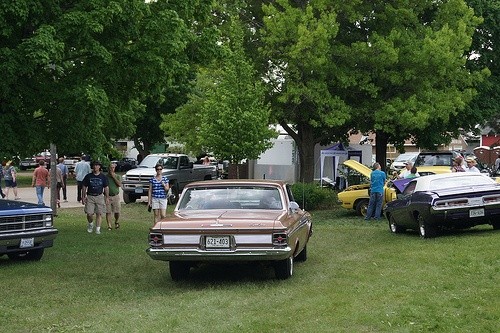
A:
95,230,100,235
108,227,112,231
115,221,119,229
15,197,21,200
87,225,93,233
63,200,68,202
57,200,60,203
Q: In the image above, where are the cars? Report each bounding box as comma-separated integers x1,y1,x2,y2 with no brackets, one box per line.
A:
337,147,500,218
0,198,58,261
146,179,313,280
19,149,138,175
383,172,500,238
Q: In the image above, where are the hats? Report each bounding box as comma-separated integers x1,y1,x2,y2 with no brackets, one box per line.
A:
404,161,413,166
91,161,103,170
452,155,463,165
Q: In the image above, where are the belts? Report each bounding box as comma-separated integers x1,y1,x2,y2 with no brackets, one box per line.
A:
109,192,119,196
88,192,102,196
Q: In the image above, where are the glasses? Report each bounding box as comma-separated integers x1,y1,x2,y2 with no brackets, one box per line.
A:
156,169,163,171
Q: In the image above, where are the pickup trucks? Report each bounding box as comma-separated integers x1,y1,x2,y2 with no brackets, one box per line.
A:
119,153,216,205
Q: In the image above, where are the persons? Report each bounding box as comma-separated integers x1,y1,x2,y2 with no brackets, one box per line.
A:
464,158,480,172
394,162,420,179
104,164,122,230
32,160,49,206
57,157,68,201
147,164,168,225
452,157,465,172
48,166,65,208
75,156,91,202
203,156,211,165
495,154,500,173
4,161,21,200
365,163,386,221
82,160,109,235
406,167,417,178
0,164,6,198
